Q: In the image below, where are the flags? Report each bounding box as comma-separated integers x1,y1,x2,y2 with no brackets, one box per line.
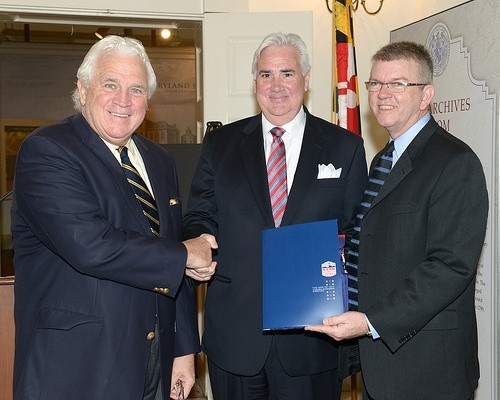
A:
332,0,363,137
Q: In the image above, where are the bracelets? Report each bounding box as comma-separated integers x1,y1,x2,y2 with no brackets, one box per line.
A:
367,327,372,336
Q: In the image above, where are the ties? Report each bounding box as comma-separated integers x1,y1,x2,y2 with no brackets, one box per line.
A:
345,140,395,311
266,127,288,229
115,146,160,238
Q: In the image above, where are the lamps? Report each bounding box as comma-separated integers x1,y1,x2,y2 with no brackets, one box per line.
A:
95,28,109,40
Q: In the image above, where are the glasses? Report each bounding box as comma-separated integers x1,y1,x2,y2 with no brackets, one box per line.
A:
364,81,430,93
174,379,185,400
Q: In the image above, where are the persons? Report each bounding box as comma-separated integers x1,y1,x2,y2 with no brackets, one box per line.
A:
185,33,369,400
11,34,213,400
304,42,489,400
